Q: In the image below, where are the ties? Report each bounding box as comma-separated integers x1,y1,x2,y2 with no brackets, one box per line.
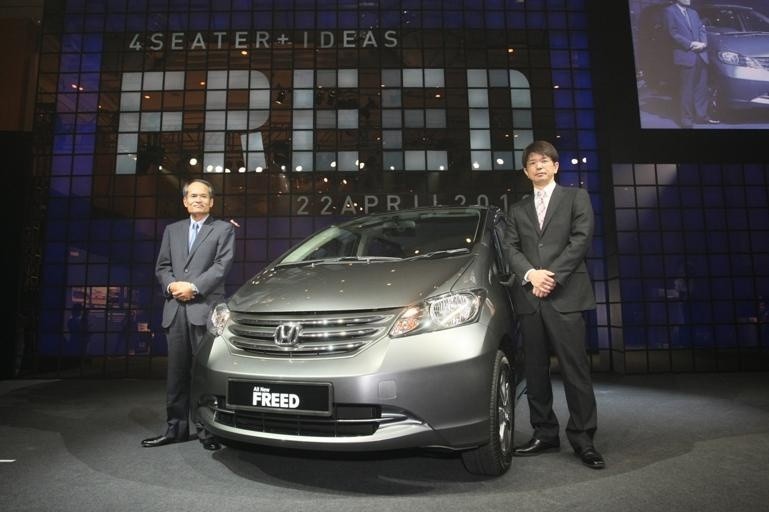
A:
189,223,198,252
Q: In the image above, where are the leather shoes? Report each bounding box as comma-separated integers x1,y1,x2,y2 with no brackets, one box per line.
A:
200,438,219,449
581,449,605,468
141,434,176,446
513,438,560,456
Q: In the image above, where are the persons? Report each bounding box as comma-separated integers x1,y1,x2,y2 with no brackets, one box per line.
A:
140,177,238,450
504,139,607,470
662,0,721,127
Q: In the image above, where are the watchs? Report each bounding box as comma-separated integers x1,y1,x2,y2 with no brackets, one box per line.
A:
191,283,197,296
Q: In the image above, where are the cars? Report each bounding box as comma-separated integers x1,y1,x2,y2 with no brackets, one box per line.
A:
640,3,769,121
190,205,530,476
